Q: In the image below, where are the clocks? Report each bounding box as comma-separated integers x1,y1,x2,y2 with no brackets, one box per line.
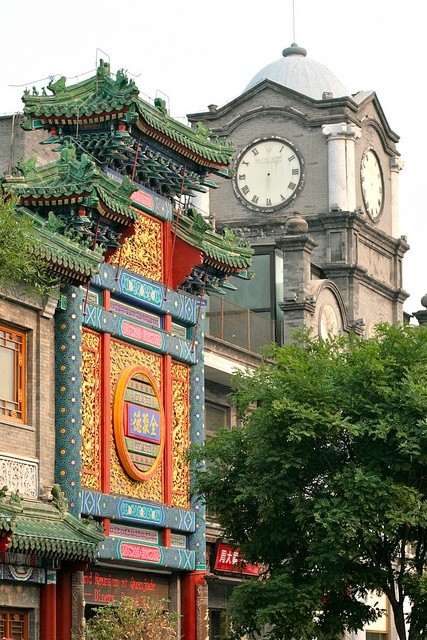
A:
229,134,306,214
361,148,385,223
318,303,340,343
